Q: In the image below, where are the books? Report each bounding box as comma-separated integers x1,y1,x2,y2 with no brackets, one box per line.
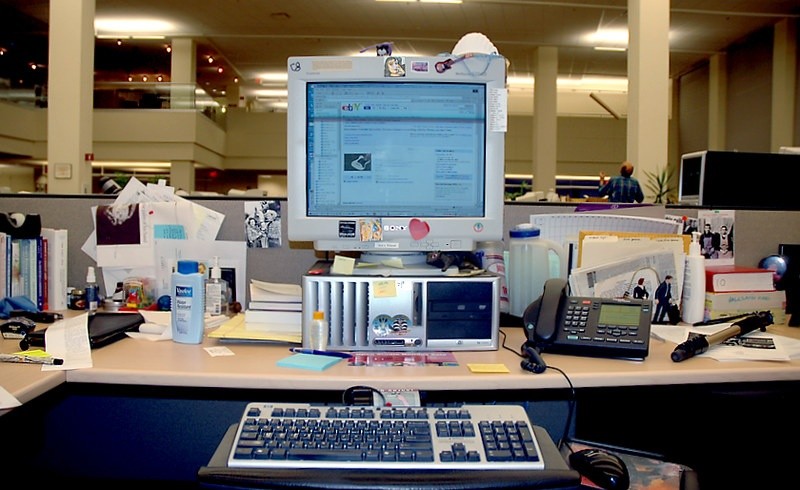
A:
245,279,302,324
706,265,792,327
0,228,67,311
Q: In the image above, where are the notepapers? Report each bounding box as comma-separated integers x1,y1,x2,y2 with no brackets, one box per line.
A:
276,353,342,371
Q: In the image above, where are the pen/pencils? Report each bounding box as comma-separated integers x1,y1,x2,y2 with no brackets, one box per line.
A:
0,353,64,365
288,347,354,359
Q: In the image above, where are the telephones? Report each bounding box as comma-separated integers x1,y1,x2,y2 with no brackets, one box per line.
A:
522,278,654,361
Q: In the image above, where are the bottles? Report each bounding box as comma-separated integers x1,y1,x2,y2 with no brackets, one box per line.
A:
67,287,75,307
70,289,86,310
308,311,329,352
84,266,99,314
474,237,510,315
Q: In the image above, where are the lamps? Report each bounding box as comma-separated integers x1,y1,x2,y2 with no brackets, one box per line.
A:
157,76,163,81
233,77,239,83
117,39,122,45
207,56,215,64
218,66,224,73
220,87,226,95
167,45,171,53
127,76,134,81
142,76,148,81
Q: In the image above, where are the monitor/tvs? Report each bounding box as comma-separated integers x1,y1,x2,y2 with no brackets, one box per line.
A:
286,55,505,276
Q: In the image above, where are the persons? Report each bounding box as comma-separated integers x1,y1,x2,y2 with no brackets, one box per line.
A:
247,207,278,248
598,162,644,203
683,220,733,259
633,275,675,324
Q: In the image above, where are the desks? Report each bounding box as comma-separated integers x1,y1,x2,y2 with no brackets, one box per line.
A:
0,292,800,490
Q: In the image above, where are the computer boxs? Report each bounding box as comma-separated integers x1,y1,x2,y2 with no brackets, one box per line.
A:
302,259,499,352
227,402,546,469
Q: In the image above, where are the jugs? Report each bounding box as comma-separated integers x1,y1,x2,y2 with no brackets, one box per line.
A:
505,224,569,318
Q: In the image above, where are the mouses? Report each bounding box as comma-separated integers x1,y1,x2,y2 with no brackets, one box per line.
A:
569,449,631,490
